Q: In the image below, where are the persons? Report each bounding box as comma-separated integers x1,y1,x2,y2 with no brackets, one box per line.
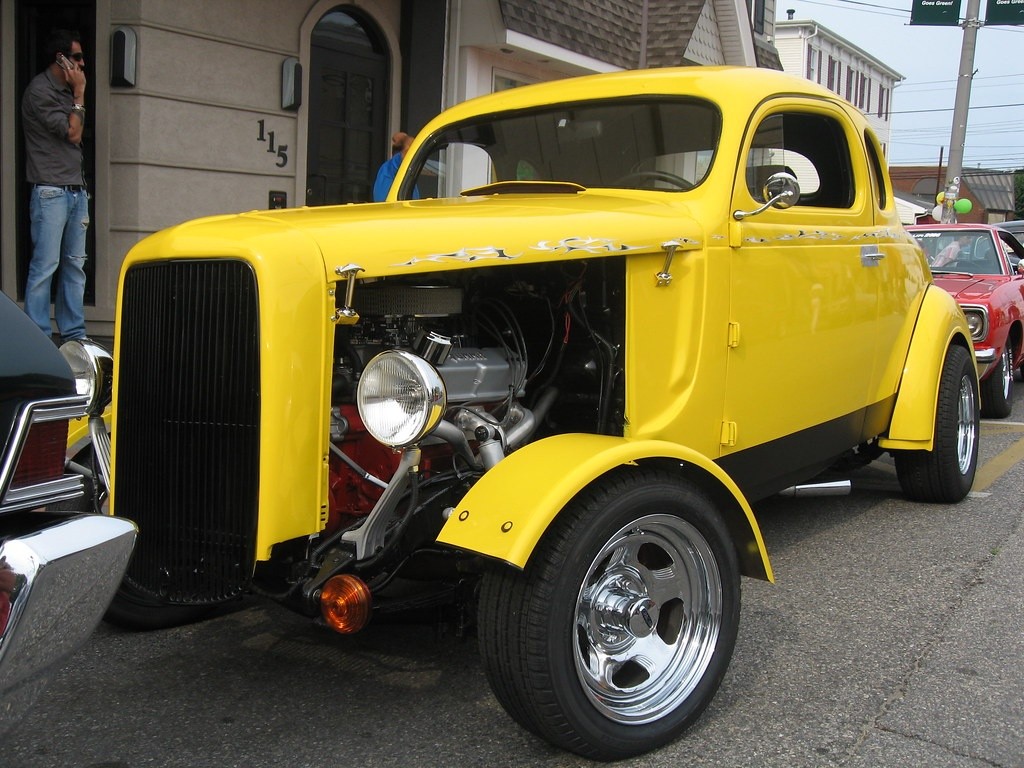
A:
372,132,419,202
930,236,971,267
21,36,91,344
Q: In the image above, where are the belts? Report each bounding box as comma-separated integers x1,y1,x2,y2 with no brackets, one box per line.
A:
36,183,89,192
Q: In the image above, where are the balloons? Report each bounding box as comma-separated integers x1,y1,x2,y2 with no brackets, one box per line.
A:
932,191,973,221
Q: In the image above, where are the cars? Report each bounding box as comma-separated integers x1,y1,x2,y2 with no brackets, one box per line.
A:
1,290,139,688
902,224,1024,418
57,65,984,762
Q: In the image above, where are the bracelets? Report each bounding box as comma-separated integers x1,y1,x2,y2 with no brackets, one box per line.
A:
72,104,85,111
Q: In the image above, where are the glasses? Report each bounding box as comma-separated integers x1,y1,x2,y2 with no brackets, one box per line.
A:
68,53,85,62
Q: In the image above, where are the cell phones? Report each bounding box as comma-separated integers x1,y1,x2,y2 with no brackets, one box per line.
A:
55,55,74,71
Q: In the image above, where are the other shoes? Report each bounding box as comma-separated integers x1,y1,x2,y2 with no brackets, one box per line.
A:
59,339,94,346
